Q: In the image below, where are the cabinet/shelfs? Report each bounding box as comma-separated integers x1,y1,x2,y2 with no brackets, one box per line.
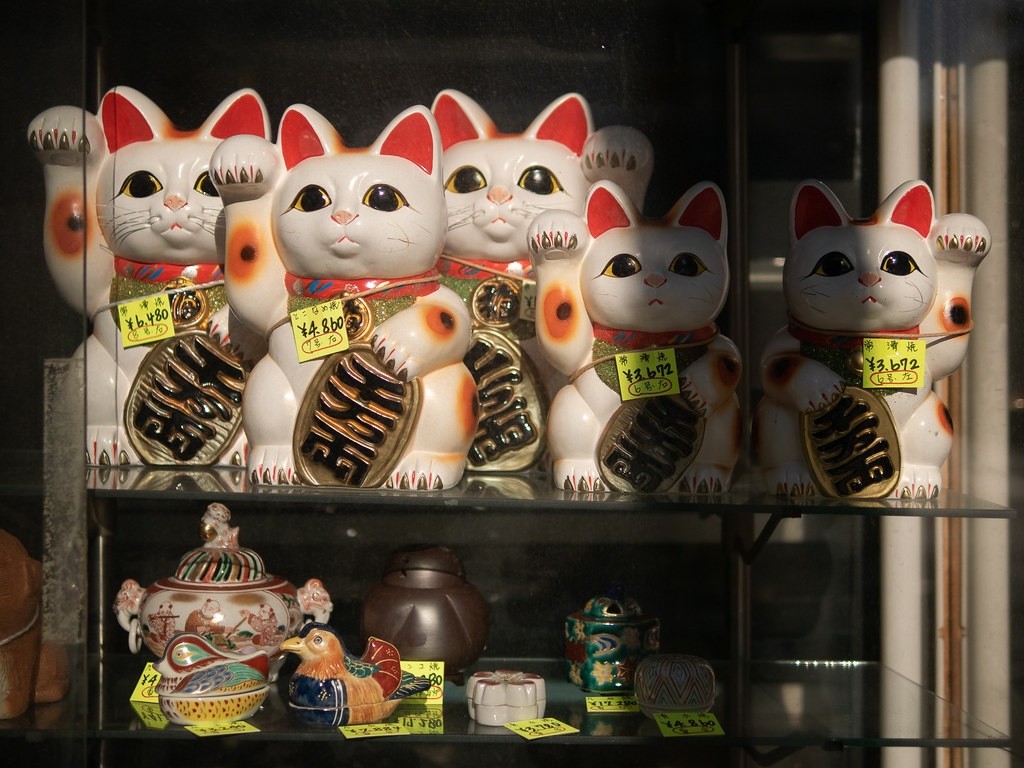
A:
79,0,1024,768
